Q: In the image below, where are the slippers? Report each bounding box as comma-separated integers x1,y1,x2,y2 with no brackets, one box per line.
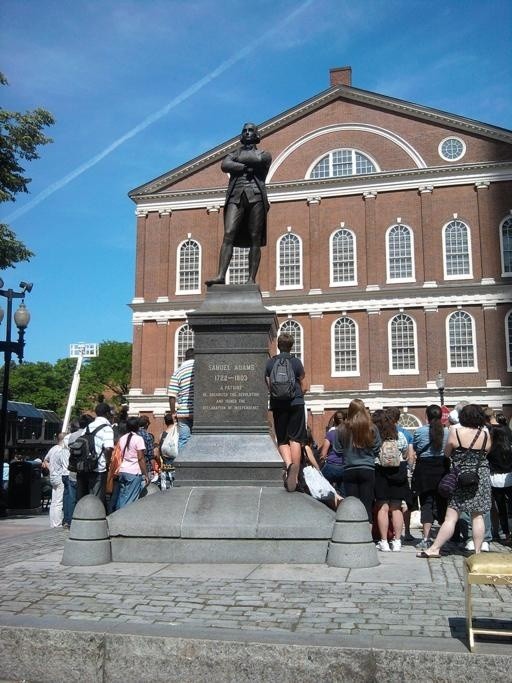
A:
416,552,442,557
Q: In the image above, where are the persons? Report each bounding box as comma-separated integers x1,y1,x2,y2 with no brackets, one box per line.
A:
33,402,179,531
205,122,273,285
167,348,195,450
297,398,512,558
266,334,308,491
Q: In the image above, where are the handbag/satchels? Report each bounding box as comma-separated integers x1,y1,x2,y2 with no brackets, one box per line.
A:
438,473,459,498
459,470,480,487
105,472,114,493
302,444,337,502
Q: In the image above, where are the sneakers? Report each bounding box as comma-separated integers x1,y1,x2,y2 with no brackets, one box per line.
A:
376,541,390,551
459,540,475,551
391,541,402,551
481,541,489,552
414,540,432,548
284,462,300,492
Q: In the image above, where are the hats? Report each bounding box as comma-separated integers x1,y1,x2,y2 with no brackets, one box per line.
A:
164,414,172,422
447,409,459,424
95,403,114,416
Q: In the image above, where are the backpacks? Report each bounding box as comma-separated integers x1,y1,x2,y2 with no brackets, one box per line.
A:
267,354,296,401
67,423,113,473
377,437,403,467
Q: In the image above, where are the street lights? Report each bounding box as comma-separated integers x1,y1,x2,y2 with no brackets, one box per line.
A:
435,371,446,427
0,277,33,518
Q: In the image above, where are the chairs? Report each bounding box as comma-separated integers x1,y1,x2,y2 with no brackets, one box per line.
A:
39,483,53,511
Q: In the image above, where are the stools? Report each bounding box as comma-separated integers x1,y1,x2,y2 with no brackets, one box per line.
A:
463,553,512,653
